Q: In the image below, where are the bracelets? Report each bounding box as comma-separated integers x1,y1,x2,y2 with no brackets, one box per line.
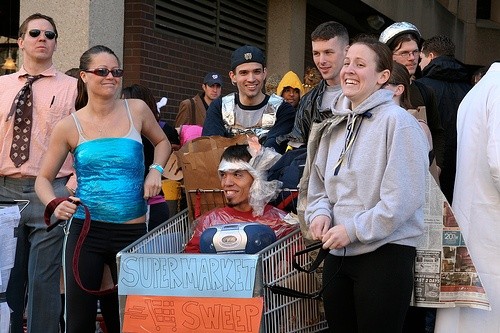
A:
148,163,164,174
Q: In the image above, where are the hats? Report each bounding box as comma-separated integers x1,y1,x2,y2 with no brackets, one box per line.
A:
203,72,223,87
231,45,266,70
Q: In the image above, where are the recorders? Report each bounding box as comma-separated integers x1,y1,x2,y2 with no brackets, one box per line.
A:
199,222,277,255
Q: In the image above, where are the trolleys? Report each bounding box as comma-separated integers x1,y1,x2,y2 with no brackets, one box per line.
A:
116,188,329,333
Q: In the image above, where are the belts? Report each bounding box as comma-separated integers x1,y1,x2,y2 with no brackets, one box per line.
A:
6,74,51,168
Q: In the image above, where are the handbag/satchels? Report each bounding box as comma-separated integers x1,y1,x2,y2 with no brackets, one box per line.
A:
179,135,254,217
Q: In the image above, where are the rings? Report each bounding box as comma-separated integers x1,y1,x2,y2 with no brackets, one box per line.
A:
66,213,69,218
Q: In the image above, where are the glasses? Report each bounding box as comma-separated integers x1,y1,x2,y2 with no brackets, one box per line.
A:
393,49,420,57
24,29,56,40
83,69,124,77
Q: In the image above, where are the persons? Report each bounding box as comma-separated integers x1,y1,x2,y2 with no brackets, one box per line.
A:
180,136,301,280
174,22,489,212
0,14,181,333
433,59,500,333
304,38,431,333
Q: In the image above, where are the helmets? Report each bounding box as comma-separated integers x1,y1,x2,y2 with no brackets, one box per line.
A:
378,21,421,46
